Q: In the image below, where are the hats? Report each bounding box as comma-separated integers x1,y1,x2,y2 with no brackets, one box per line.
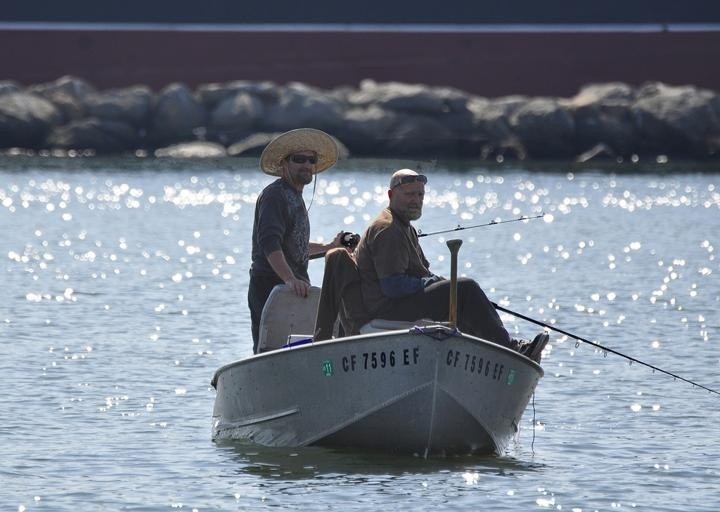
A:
258,126,339,175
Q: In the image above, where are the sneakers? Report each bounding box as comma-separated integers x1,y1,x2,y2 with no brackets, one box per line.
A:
519,332,549,364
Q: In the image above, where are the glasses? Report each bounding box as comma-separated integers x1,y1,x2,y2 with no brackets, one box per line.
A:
393,174,428,190
283,154,316,163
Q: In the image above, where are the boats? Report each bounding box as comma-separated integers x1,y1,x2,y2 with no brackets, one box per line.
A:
209,324,545,459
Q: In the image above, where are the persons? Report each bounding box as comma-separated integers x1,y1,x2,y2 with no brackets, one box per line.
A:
248,127,360,353
352,169,549,366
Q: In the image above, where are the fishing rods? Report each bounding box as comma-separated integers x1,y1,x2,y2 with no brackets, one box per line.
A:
340,211,551,252
488,301,720,395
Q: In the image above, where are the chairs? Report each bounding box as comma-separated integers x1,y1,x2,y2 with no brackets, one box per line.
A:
352,319,413,334
256,285,321,353
